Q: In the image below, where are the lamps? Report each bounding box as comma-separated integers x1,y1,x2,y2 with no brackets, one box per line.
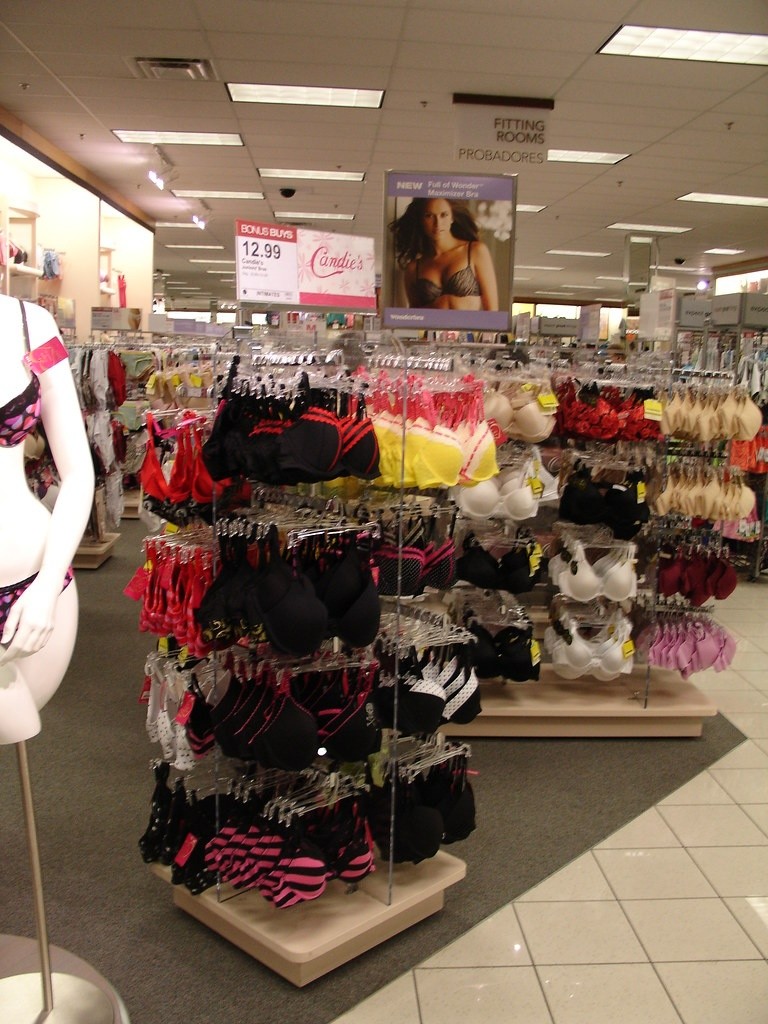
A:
149,164,172,180
196,215,216,229
155,169,179,190
192,210,210,223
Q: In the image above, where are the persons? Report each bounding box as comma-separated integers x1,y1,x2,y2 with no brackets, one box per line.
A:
389,198,499,313
0,295,95,748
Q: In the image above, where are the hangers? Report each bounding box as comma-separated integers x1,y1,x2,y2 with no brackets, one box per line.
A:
68,341,768,828
